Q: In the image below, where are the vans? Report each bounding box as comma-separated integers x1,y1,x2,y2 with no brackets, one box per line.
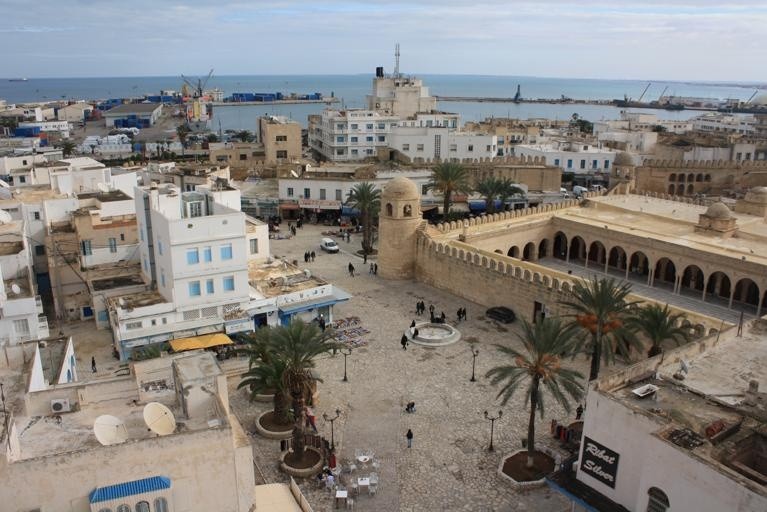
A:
320,238,339,253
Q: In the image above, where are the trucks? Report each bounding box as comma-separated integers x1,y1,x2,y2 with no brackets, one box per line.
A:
573,186,588,196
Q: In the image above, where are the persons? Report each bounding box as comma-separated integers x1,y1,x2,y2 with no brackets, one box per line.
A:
304,250,316,261
287,213,304,236
400,301,467,349
407,429,413,447
406,402,414,411
92,356,96,372
369,262,377,275
576,404,583,420
348,262,356,278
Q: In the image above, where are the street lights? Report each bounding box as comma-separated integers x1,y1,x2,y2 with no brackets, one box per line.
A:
470,344,480,381
483,410,503,450
339,348,353,381
323,409,340,451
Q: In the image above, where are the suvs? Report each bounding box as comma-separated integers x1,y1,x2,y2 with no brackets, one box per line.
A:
486,306,515,324
